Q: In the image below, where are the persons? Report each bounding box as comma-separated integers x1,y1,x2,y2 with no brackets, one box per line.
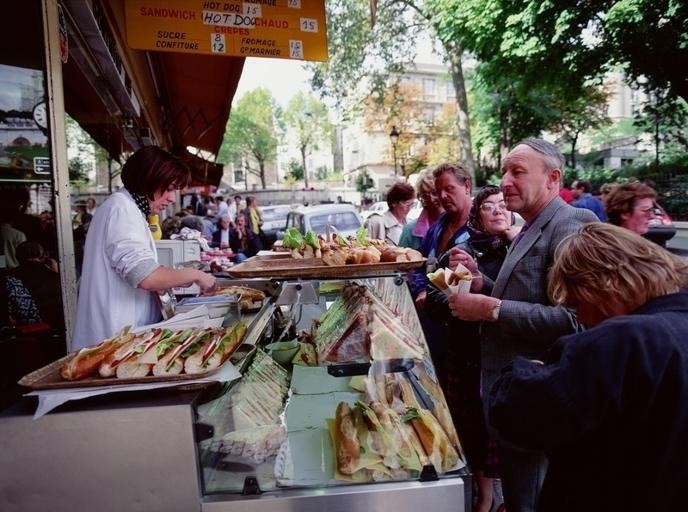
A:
234,196,246,219
450,138,599,512
426,185,520,511
209,196,228,223
570,180,609,223
560,181,574,203
369,183,415,246
72,200,92,233
0,205,27,275
408,164,515,512
235,214,254,258
211,215,246,263
482,224,688,512
243,196,268,256
605,182,655,236
397,169,446,250
70,146,217,352
227,198,236,220
0,241,65,337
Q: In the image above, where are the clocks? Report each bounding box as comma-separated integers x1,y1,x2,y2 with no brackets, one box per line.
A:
32,100,48,136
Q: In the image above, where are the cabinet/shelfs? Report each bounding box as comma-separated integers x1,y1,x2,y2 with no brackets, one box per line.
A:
0,275,472,512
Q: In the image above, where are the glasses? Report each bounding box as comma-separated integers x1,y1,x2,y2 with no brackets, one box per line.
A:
418,191,442,201
481,203,505,210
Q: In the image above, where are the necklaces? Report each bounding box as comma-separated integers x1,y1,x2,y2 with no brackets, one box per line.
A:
448,218,468,240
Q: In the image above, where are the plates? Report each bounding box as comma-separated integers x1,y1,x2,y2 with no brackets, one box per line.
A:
274,389,463,487
291,366,352,394
228,247,425,276
18,322,248,388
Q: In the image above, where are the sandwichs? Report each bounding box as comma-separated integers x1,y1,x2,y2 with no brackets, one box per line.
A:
313,281,425,363
448,272,470,288
60,325,136,380
334,398,361,476
150,325,216,376
428,269,447,290
198,349,289,462
94,327,161,376
118,327,182,378
185,316,247,375
365,371,464,476
271,228,422,267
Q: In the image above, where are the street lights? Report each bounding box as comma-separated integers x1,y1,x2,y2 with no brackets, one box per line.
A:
390,125,399,175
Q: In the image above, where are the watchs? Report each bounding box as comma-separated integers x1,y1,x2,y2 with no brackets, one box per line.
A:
493,300,501,320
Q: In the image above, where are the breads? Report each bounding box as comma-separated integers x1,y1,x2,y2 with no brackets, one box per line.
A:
216,285,265,310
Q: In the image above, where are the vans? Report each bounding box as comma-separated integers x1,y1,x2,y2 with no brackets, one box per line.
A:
259,204,304,229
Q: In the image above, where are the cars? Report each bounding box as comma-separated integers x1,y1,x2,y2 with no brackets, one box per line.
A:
591,195,676,250
285,204,362,237
361,201,388,229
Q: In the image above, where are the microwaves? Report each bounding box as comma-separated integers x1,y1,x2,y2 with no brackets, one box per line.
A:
156,240,200,295
